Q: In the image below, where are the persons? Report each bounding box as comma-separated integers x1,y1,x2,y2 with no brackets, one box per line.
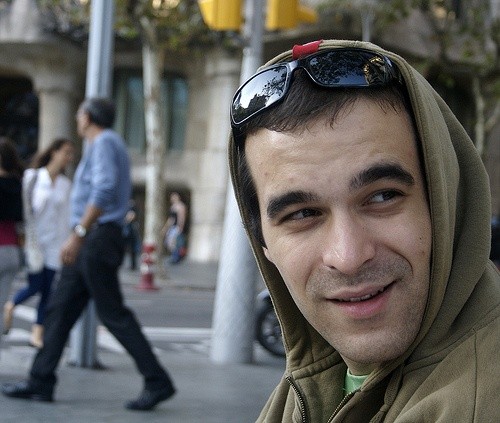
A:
0,97,176,410
229,37,499,423
159,191,188,265
117,194,137,270
3,136,75,347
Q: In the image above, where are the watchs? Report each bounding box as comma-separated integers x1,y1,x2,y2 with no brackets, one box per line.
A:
72,224,89,239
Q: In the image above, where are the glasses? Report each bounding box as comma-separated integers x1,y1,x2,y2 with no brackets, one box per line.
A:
230,47,403,125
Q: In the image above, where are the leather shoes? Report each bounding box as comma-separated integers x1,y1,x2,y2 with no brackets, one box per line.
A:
2,379,54,401
127,381,175,410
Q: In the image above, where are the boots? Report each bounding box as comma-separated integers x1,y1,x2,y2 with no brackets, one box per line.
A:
29,324,44,349
3,299,14,334
